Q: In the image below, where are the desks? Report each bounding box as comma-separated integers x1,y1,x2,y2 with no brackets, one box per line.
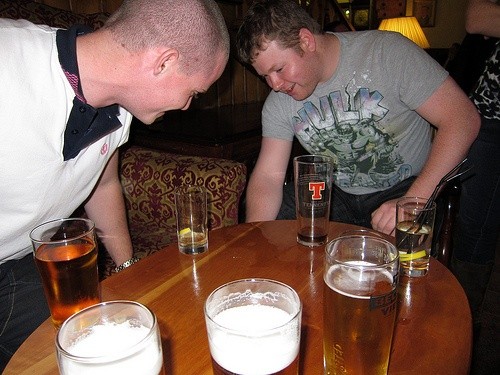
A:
0,220,472,375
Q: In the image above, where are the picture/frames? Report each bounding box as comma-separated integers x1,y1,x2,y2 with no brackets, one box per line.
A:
412,0,437,28
371,0,407,30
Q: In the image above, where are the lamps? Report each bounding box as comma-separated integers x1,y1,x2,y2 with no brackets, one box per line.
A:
377,16,430,49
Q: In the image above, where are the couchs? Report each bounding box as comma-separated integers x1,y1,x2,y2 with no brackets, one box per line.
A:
0,0,247,282
416,48,500,142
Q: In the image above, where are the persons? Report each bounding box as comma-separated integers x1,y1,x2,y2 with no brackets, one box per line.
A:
0,0,231,375
233,0,482,253
445,1,500,375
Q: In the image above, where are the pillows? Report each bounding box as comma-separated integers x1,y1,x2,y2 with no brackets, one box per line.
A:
450,33,500,134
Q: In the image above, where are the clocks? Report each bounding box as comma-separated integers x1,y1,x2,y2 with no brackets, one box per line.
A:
353,9,368,26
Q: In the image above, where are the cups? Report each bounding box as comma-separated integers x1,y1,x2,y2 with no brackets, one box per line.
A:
175,185,209,256
293,154,334,248
338,228,392,246
55,300,165,375
322,235,400,375
29,218,102,331
395,197,436,278
204,279,304,375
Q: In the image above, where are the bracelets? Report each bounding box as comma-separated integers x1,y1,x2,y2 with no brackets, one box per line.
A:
114,257,141,273
